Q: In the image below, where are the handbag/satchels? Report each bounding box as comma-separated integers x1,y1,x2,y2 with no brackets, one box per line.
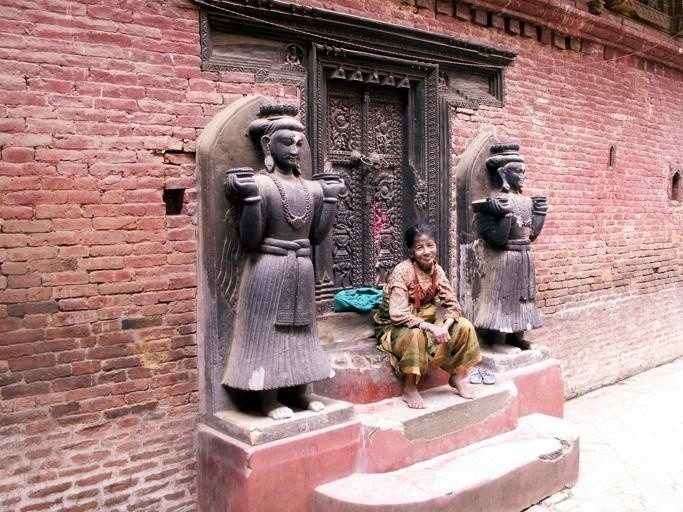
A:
334,288,383,313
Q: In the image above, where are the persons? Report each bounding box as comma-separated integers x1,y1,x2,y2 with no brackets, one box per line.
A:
373,221,484,410
223,103,346,421
472,141,548,355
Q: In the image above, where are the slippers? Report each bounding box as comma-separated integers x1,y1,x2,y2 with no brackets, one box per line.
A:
469,368,495,384
